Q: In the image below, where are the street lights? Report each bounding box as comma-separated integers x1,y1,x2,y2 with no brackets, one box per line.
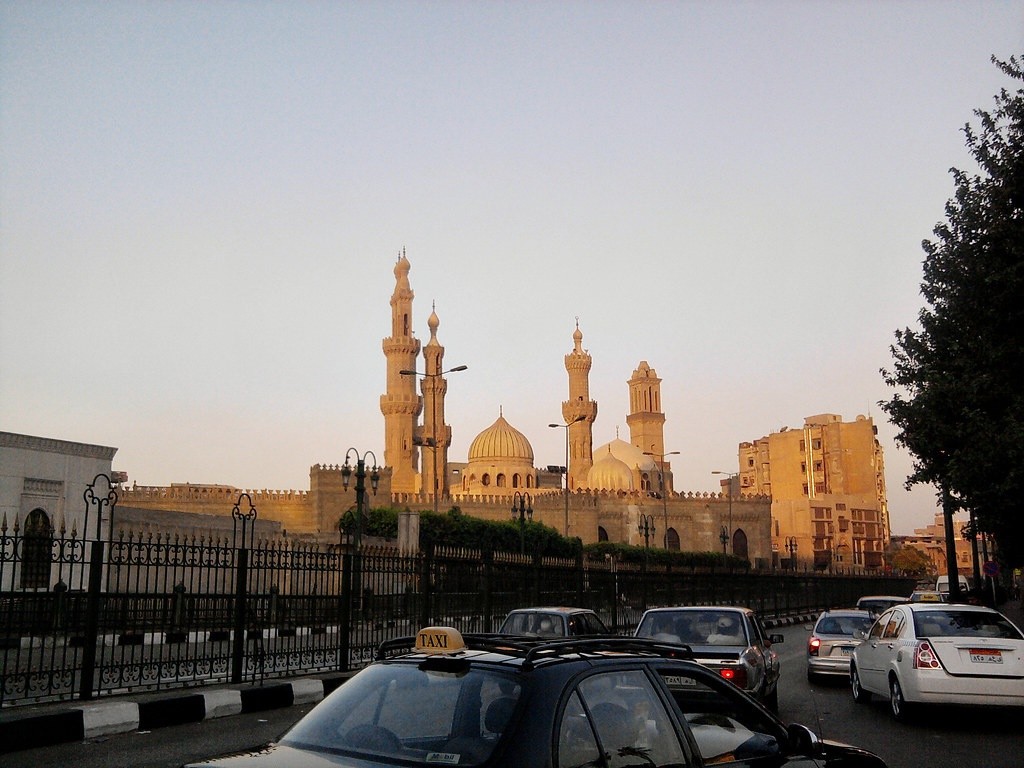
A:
719,525,729,569
643,452,680,550
785,536,798,571
638,514,655,572
511,492,534,554
549,415,587,538
342,448,379,631
712,471,749,555
400,366,467,512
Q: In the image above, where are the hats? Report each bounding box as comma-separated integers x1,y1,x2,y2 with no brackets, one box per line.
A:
718,616,733,627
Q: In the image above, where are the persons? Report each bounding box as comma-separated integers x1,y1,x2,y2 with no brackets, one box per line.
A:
684,712,737,733
652,613,681,642
706,617,742,646
583,703,638,752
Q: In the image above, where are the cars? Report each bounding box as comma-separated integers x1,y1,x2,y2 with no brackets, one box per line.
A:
174,626,889,768
851,591,1024,722
856,596,910,616
614,606,784,715
804,607,882,685
498,607,610,637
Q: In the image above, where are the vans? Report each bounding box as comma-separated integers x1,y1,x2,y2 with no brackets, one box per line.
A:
937,575,969,593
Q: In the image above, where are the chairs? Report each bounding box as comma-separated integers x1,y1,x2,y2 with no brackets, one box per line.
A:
564,701,646,768
345,725,404,754
485,695,519,735
444,734,493,766
